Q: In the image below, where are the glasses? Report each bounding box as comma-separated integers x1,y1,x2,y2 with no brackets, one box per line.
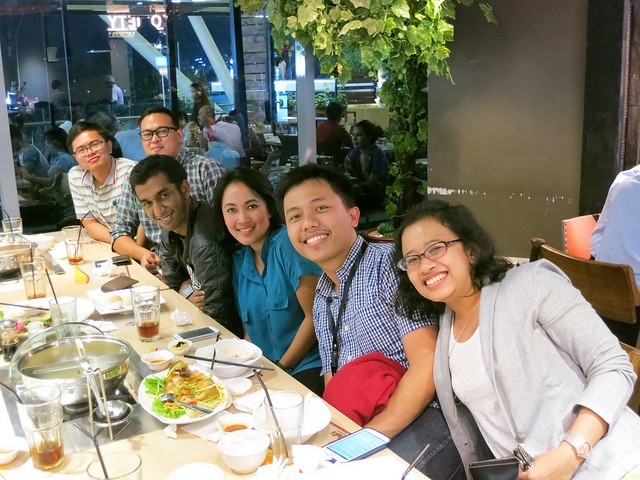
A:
396,238,464,271
513,445,534,470
137,126,177,141
73,140,107,156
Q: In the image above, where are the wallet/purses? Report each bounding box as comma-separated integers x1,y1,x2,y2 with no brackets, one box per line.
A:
469,456,520,480
101,275,139,293
112,254,132,266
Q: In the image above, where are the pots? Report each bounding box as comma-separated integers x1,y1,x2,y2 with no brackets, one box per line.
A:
12,334,133,407
0,240,39,275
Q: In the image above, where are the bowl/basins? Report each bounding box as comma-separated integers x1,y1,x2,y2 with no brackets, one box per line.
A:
0,441,18,465
217,426,271,474
167,339,194,354
252,387,333,444
218,414,253,431
194,336,271,378
215,338,258,362
140,349,174,371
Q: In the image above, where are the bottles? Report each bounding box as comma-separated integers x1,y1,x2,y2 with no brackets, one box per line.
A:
25,322,46,348
0,330,18,361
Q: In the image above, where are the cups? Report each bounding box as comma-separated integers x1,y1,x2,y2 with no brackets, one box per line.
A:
2,217,24,242
87,450,142,480
14,385,64,471
18,256,50,299
62,225,84,264
264,391,305,444
49,297,82,344
129,286,162,344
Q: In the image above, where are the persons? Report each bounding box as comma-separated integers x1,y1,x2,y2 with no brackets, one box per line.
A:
9,124,50,177
50,79,69,107
129,154,244,339
316,101,354,155
229,108,263,156
104,75,124,105
20,128,79,187
179,109,188,129
343,119,390,198
108,107,225,274
213,166,324,399
189,83,210,131
67,122,151,250
391,199,640,480
197,104,217,141
278,163,439,447
589,163,640,292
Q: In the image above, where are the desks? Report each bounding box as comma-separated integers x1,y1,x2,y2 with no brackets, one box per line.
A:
23,114,143,127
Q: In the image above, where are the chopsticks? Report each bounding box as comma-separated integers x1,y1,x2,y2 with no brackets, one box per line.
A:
0,268,68,317
183,332,274,378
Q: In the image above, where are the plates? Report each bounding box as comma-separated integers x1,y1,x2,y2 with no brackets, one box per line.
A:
1,436,29,469
188,360,266,380
3,295,94,325
314,455,415,480
169,462,223,479
87,283,167,317
136,366,232,426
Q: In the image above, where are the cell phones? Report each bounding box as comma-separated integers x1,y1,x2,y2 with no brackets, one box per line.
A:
172,325,220,343
321,427,391,463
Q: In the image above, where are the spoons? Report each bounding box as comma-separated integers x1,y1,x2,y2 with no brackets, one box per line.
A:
82,358,130,419
157,392,214,415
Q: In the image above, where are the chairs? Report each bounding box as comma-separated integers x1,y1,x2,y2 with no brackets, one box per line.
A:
530,236,639,370
559,212,600,260
249,126,265,151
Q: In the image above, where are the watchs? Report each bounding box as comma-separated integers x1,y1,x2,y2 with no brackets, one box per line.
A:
561,433,592,459
